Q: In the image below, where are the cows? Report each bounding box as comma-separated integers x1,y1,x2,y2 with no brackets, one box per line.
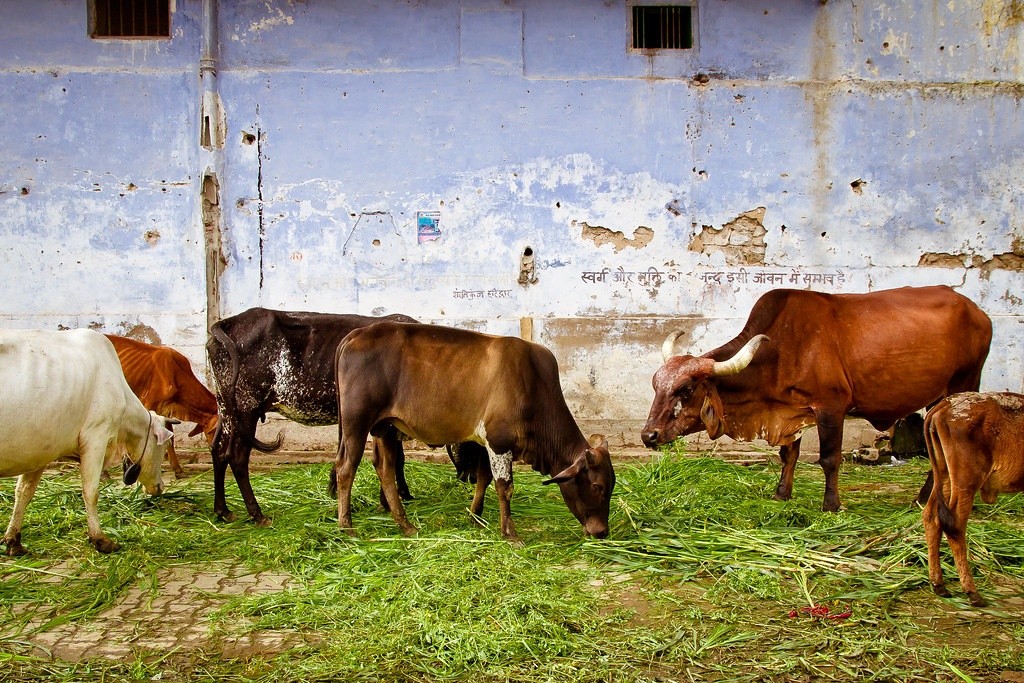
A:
641,284,993,512
921,390,1024,607
328,319,616,542
0,328,221,557
206,307,478,525
